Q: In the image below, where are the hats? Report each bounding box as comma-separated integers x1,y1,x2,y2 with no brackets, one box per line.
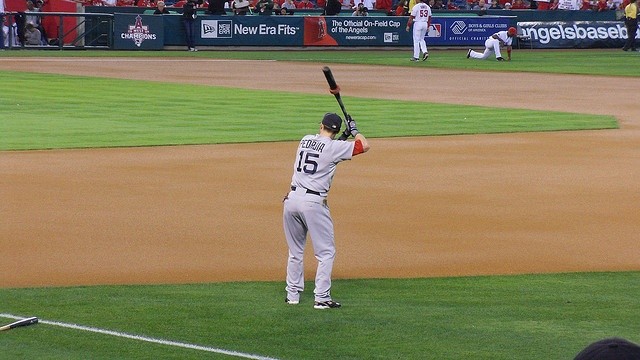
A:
508,27,516,34
505,3,511,6
574,337,640,359
323,112,342,129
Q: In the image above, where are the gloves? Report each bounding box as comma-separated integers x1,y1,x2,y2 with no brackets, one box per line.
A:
344,118,358,137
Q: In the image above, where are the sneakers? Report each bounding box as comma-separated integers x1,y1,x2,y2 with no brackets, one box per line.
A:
195,49,197,51
285,297,299,304
422,52,429,61
496,57,504,61
622,47,627,51
631,49,639,51
410,57,419,62
467,49,472,58
188,47,194,51
313,300,342,309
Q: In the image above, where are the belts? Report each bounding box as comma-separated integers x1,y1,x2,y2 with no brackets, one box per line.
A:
338,128,351,141
291,186,320,196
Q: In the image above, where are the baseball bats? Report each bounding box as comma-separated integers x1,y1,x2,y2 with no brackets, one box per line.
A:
323,66,349,122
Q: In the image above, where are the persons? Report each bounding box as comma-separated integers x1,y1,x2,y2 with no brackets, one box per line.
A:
26,23,40,43
282,113,371,310
183,0,198,53
511,0,531,9
324,1,341,14
549,0,584,10
254,1,274,15
154,1,170,14
282,0,296,7
234,1,249,15
25,1,39,22
474,1,510,10
622,0,639,51
353,3,369,16
278,7,288,14
406,0,431,62
466,27,517,62
300,0,313,8
